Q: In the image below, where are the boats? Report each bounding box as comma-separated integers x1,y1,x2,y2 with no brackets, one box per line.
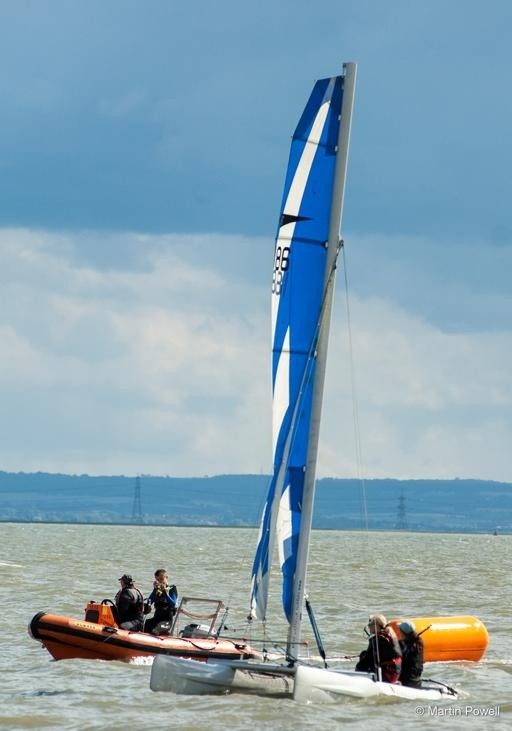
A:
27,595,263,668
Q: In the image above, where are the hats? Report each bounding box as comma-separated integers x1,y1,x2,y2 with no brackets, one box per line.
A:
396,621,414,634
118,573,134,582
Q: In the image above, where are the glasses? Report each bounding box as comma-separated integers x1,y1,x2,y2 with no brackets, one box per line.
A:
367,622,376,628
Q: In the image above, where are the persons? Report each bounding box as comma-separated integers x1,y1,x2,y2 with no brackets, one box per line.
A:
144,568,178,633
355,614,404,684
115,574,144,632
397,620,423,688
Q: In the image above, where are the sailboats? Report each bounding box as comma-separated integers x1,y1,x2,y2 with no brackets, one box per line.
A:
148,58,458,709
